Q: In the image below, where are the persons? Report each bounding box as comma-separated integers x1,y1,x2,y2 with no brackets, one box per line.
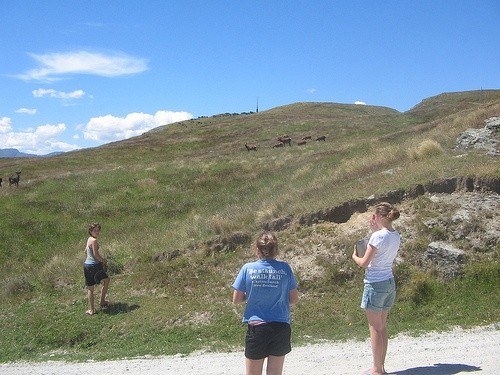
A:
83,223,110,316
352,202,400,375
232,233,298,375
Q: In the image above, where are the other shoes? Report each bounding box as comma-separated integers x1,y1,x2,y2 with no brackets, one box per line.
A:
86,310,94,315
100,301,108,306
